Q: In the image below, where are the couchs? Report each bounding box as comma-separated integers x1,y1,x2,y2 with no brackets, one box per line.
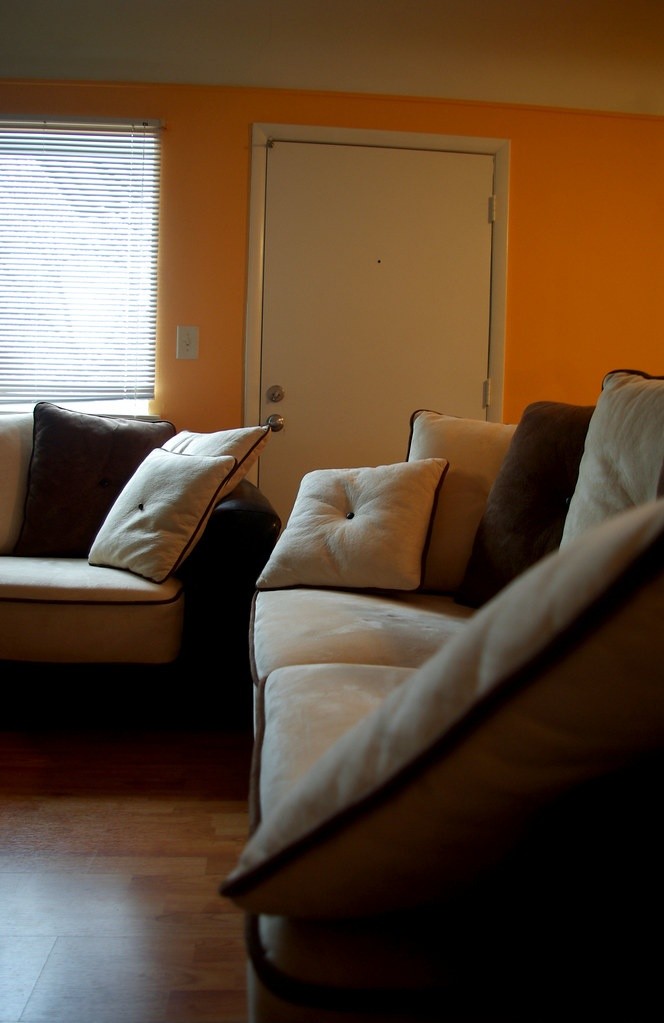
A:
0,396,283,741
220,369,664,1023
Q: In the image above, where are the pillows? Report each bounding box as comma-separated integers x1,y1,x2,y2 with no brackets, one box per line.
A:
224,493,664,936
87,449,237,586
160,423,272,508
450,400,599,609
255,456,451,596
560,368,664,557
404,402,520,596
0,400,177,560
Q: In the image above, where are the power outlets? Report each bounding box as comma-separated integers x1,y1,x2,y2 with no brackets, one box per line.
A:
176,325,199,360
